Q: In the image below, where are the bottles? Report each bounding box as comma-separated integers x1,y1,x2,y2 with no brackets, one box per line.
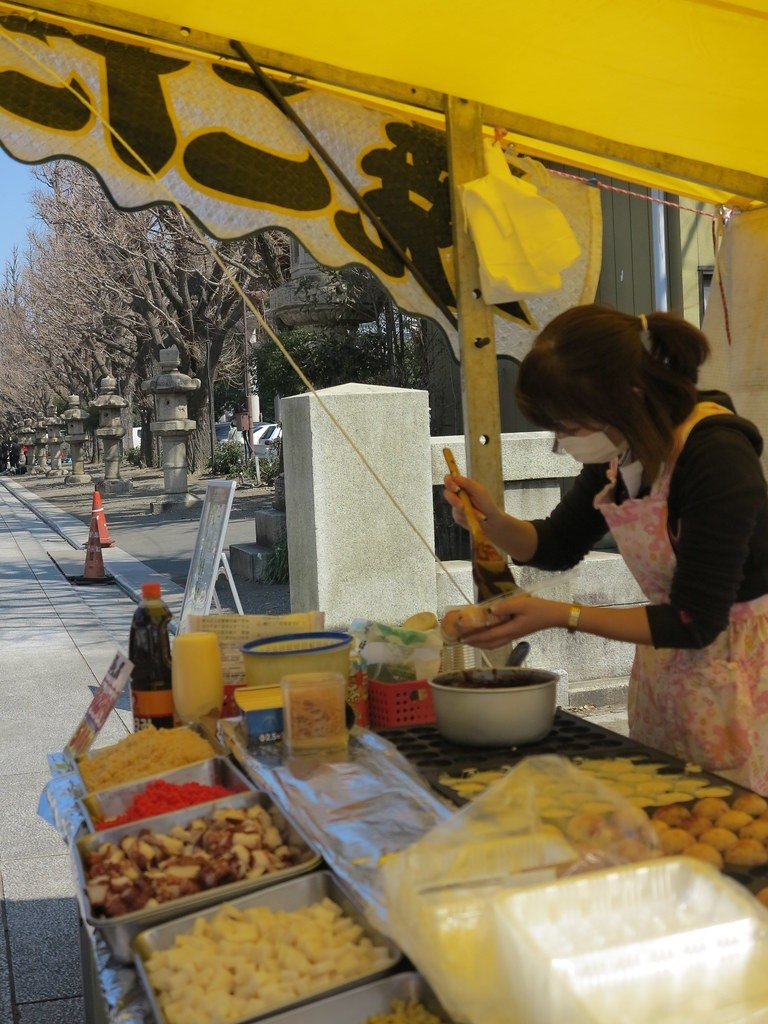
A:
128,584,180,729
279,671,350,750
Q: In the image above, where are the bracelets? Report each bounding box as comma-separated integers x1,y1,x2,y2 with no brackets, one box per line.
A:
569,603,581,634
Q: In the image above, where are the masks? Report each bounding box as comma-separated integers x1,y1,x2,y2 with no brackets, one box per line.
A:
557,423,628,464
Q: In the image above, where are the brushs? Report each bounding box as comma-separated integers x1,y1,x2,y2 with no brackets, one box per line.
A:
442,448,520,602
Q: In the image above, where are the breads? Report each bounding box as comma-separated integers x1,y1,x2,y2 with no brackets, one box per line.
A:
542,792,768,877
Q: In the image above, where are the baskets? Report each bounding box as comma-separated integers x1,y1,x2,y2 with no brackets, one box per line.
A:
367,680,436,728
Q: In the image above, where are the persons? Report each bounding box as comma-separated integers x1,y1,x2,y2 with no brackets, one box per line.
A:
443,305,768,797
0,437,21,475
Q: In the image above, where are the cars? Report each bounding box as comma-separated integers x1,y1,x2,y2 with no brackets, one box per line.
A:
211,421,283,448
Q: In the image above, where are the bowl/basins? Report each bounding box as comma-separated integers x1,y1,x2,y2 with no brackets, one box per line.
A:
434,566,580,643
493,851,768,1024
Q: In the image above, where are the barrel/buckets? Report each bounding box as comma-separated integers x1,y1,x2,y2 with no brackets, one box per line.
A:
240,633,353,691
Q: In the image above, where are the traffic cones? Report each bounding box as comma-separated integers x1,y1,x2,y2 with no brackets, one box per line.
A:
68,516,116,584
80,490,115,548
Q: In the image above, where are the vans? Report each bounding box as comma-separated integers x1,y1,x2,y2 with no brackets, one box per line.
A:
133,426,142,450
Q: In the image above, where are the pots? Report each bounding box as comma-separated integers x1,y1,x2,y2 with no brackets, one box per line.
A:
425,640,560,746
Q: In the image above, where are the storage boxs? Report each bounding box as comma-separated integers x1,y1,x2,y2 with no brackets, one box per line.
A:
69,610,468,1024
488,849,768,1024
